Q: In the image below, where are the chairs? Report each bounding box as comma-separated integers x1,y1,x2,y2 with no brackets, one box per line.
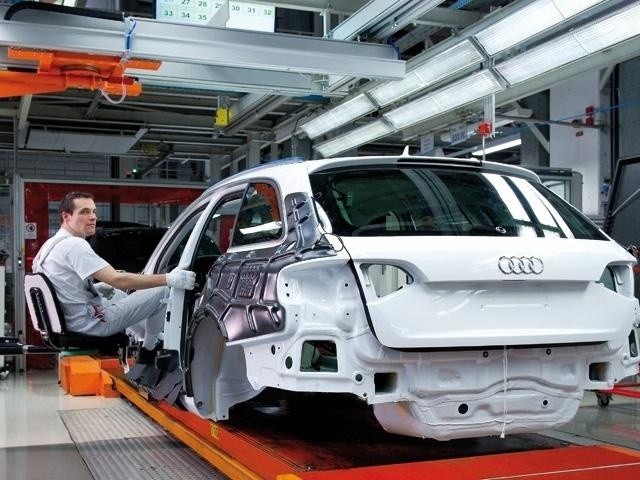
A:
23,272,130,353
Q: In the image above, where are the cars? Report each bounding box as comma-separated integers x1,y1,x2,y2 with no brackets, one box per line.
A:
116,147,640,445
85,219,223,331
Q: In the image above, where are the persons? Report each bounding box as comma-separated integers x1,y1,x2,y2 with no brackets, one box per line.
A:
30,189,200,366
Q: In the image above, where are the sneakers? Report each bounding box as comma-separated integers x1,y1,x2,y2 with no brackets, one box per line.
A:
135,336,163,366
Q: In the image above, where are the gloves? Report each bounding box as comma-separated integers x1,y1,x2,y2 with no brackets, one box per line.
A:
93,282,115,300
165,265,196,290
